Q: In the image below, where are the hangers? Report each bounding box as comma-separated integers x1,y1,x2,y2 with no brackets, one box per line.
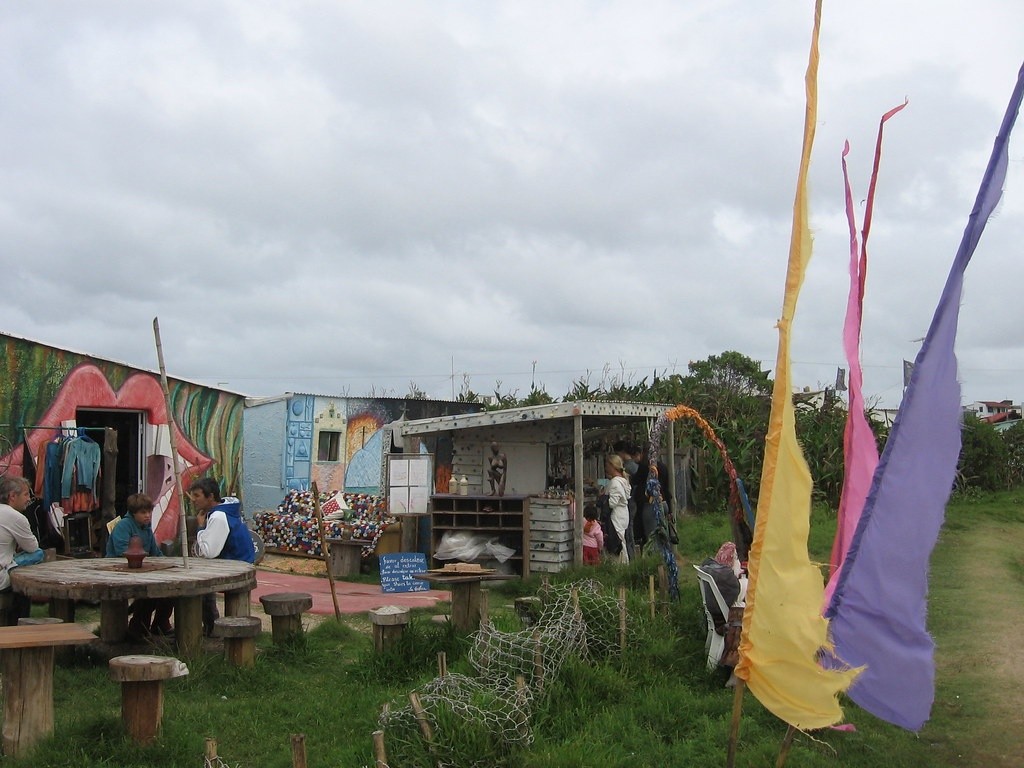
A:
40,427,94,444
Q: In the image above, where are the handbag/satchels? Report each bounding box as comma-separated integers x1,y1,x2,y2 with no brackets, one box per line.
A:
668,523,679,545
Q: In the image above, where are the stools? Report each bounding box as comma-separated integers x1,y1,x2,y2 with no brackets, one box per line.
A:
214,616,262,667
259,593,312,645
515,597,541,617
110,655,189,744
369,605,410,652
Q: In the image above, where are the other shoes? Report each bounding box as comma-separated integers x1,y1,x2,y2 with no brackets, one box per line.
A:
156,628,176,638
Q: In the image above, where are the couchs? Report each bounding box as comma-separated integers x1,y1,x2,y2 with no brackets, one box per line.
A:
252,489,403,559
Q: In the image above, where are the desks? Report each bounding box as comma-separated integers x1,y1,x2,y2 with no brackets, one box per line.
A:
10,556,257,668
0,624,98,758
415,572,520,623
326,539,371,576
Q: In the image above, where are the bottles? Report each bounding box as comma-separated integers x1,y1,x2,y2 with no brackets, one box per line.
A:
460,475,468,495
449,475,457,494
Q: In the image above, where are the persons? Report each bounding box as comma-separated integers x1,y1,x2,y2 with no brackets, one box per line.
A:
104,492,175,637
567,493,603,565
600,455,631,566
0,477,45,593
487,441,507,497
192,478,255,638
614,441,672,554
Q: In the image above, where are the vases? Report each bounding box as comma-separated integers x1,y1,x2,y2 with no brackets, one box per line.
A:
341,508,355,539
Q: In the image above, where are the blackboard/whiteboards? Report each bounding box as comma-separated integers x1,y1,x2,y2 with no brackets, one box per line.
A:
378,553,430,593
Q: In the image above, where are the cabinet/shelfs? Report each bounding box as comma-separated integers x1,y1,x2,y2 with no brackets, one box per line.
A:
429,494,539,576
